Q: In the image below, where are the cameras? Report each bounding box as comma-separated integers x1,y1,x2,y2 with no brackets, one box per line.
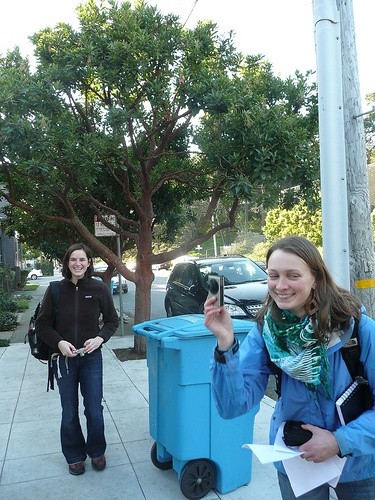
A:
206,275,225,314
73,347,87,357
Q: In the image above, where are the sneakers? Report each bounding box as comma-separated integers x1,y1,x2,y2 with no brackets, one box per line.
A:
91,455,106,470
69,461,85,475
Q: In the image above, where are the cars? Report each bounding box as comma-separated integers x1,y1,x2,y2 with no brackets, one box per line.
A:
152,262,170,271
253,260,268,274
91,266,129,295
126,261,136,272
26,268,44,280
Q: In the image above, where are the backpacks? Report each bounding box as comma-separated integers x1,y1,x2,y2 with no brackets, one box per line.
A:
28,281,61,361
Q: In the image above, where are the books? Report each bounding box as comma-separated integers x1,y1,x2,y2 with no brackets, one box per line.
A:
334,377,375,427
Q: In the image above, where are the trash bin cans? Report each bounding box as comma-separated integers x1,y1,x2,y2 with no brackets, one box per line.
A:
132,313,260,500
97,278,114,295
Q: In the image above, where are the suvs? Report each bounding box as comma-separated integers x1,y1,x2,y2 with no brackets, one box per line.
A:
163,253,270,320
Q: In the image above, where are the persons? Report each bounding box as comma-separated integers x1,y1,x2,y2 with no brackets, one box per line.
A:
205,235,375,500
34,243,118,474
